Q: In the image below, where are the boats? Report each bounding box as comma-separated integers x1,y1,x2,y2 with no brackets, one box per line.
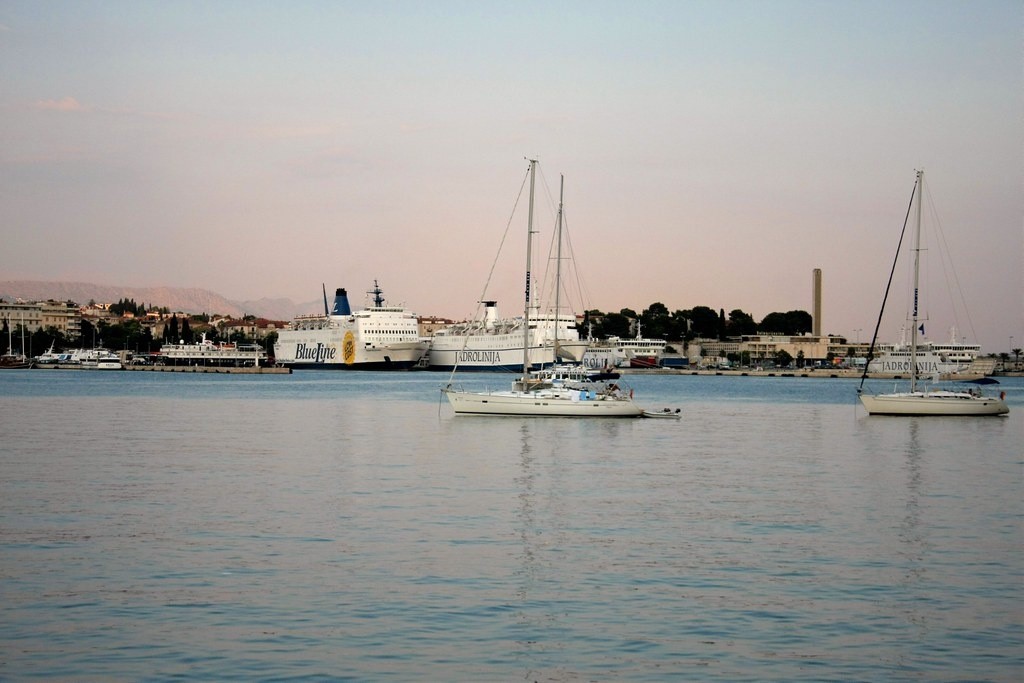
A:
641,407,682,419
0,173,691,372
867,319,997,376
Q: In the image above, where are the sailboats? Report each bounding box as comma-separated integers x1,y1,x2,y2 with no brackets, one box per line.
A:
853,167,1012,416
435,157,647,417
